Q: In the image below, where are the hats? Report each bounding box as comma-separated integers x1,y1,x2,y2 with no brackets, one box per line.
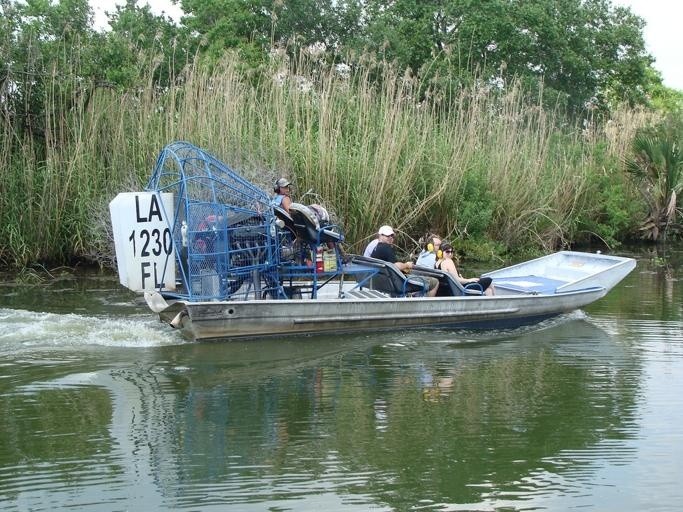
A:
378,225,395,237
273,178,293,188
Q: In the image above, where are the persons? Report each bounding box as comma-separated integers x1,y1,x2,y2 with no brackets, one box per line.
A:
270,178,292,219
363,228,396,259
415,232,433,253
415,234,443,270
369,224,440,297
433,241,495,296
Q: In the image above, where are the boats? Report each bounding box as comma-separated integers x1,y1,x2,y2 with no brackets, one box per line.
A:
108,141,638,341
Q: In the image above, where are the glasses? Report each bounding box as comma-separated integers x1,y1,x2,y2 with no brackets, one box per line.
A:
445,250,454,253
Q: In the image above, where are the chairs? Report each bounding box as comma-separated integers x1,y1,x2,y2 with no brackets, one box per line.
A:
402,260,482,295
344,252,429,297
